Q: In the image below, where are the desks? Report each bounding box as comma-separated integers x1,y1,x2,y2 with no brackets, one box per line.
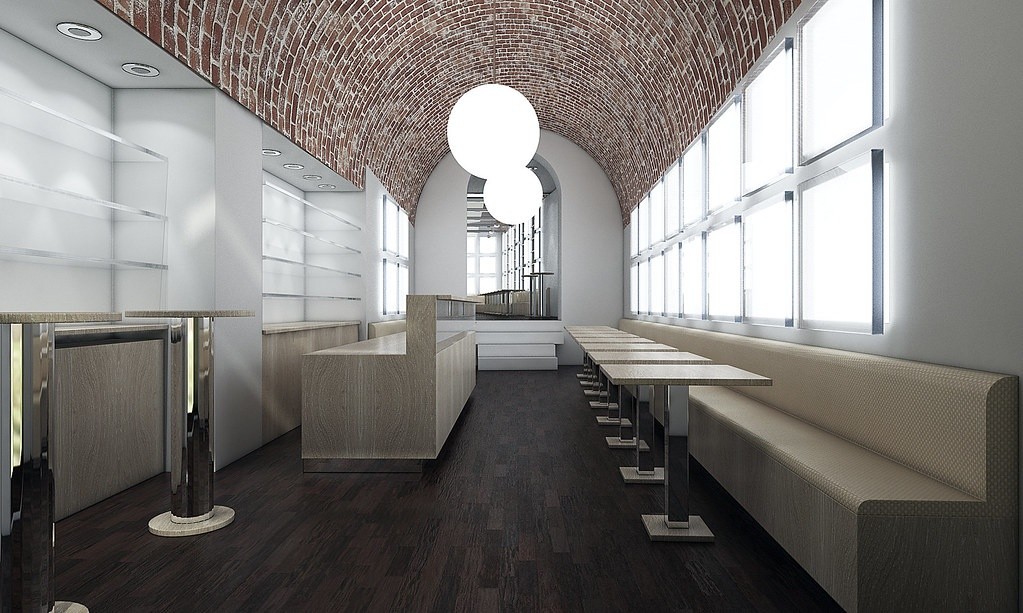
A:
564,325,772,542
262,322,360,442
478,271,554,318
0,307,255,613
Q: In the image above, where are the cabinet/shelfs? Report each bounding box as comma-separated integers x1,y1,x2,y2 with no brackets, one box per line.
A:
0,87,169,271
300,294,481,472
261,181,361,301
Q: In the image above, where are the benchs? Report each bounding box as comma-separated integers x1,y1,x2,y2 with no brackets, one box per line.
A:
619,318,1020,613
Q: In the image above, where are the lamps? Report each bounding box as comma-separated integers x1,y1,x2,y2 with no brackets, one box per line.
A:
447,0,543,225
